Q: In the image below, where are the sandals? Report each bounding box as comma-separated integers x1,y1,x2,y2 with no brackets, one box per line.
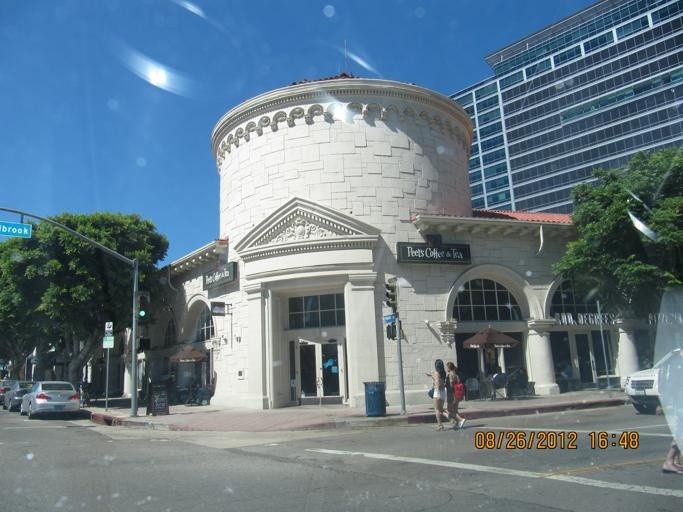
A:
662,462,683,475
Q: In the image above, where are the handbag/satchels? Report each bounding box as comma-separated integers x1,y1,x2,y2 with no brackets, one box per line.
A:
427,388,434,398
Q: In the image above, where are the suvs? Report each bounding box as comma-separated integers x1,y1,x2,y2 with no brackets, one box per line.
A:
624,346,682,414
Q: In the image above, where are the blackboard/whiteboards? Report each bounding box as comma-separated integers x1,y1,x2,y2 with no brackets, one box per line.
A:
150,383,169,416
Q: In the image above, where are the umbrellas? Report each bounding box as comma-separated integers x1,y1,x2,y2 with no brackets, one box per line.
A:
168,345,207,407
461,323,520,372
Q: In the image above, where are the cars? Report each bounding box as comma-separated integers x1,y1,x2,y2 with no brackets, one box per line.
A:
0,378,18,405
19,380,79,419
2,380,36,412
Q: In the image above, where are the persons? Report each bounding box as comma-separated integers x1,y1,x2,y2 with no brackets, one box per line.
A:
662,438,682,475
508,368,528,398
489,367,501,400
446,363,466,428
424,359,456,430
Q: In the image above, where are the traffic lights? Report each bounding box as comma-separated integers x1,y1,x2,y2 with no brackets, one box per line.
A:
137,293,150,321
384,278,397,312
386,323,398,340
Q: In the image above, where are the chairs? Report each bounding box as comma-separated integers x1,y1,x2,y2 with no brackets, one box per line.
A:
461,368,523,400
145,377,213,407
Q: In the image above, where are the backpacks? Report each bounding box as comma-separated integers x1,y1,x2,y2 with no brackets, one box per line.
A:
447,374,464,399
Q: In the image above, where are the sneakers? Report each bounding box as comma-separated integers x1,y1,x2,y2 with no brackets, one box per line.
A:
436,425,443,431
459,418,466,429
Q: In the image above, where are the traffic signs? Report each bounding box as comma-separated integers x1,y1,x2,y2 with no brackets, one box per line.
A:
0,221,31,238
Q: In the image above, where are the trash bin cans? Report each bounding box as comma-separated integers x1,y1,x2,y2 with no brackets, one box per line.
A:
363,382,387,417
